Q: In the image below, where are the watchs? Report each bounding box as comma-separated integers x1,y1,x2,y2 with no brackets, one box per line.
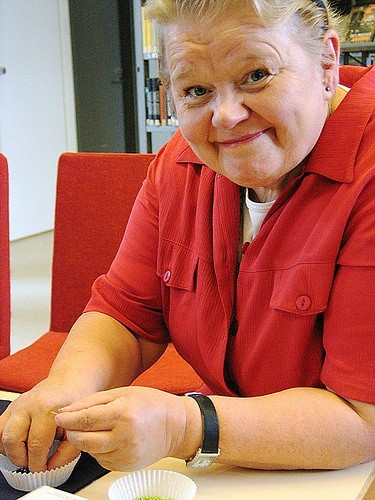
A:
184,392,220,473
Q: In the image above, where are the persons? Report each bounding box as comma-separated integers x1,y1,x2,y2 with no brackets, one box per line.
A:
0,1,373,474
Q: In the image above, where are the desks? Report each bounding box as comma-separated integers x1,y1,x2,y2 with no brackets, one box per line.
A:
0,390,375,500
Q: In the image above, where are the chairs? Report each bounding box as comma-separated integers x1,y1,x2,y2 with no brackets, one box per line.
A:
0,152,204,397
